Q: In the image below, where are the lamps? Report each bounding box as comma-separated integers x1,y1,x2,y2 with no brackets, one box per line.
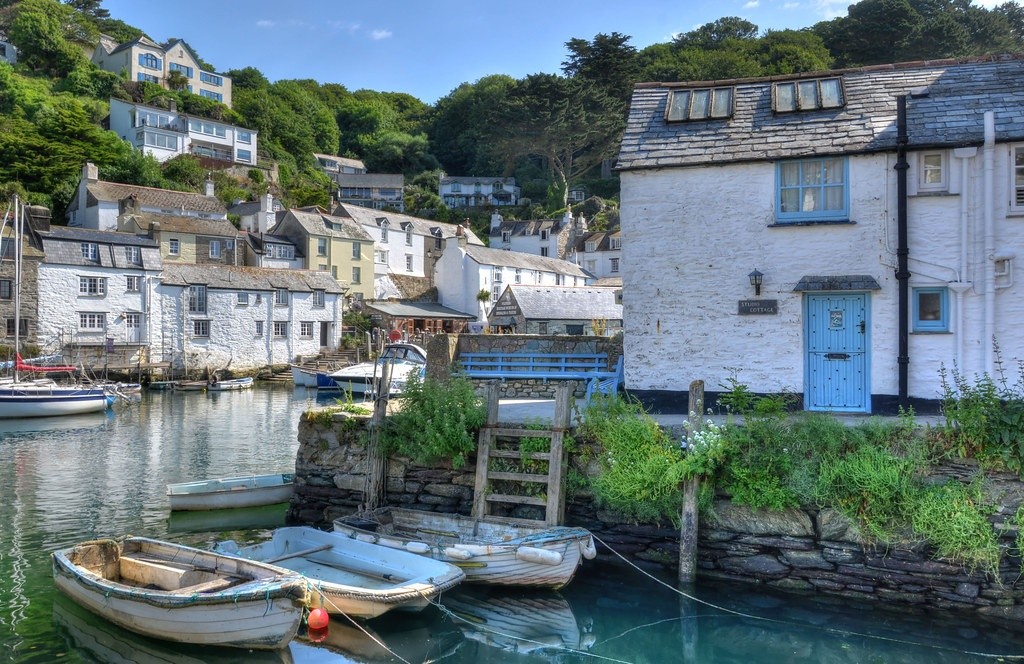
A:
748,268,764,295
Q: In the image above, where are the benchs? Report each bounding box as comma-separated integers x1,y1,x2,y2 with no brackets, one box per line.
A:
449,353,619,385
586,355,624,408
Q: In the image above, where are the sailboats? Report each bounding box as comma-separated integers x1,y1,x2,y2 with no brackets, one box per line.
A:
0,194,142,417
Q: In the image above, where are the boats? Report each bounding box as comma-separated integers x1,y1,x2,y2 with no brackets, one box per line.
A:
329,344,427,394
217,526,465,620
147,381,175,389
207,377,254,390
316,373,340,388
174,380,208,390
51,534,306,648
300,371,315,387
166,474,296,512
333,506,597,591
290,363,303,385
